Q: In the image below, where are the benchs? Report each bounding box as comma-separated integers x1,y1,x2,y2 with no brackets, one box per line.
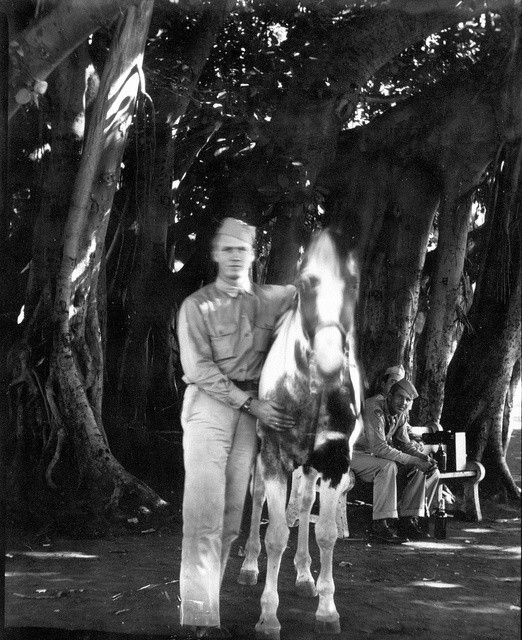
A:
423,461,487,524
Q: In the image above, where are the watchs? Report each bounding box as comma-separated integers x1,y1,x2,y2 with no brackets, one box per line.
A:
243,401,251,410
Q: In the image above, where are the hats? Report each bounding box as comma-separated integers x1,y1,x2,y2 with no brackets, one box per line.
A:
396,377,420,400
384,364,406,378
216,215,257,246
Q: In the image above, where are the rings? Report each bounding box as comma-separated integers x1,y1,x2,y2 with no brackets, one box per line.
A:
275,422,278,426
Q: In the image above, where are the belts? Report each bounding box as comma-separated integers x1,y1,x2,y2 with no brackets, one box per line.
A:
234,379,259,391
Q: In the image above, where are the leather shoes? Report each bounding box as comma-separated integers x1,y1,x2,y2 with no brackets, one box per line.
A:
366,525,410,543
194,625,226,640
397,521,432,539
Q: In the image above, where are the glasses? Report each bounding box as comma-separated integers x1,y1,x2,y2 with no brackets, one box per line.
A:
385,380,397,386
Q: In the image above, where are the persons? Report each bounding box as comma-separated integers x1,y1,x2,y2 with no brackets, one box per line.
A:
376,364,440,533
176,215,296,640
351,378,438,543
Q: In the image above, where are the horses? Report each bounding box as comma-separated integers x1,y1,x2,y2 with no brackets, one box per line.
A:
234,205,372,639
